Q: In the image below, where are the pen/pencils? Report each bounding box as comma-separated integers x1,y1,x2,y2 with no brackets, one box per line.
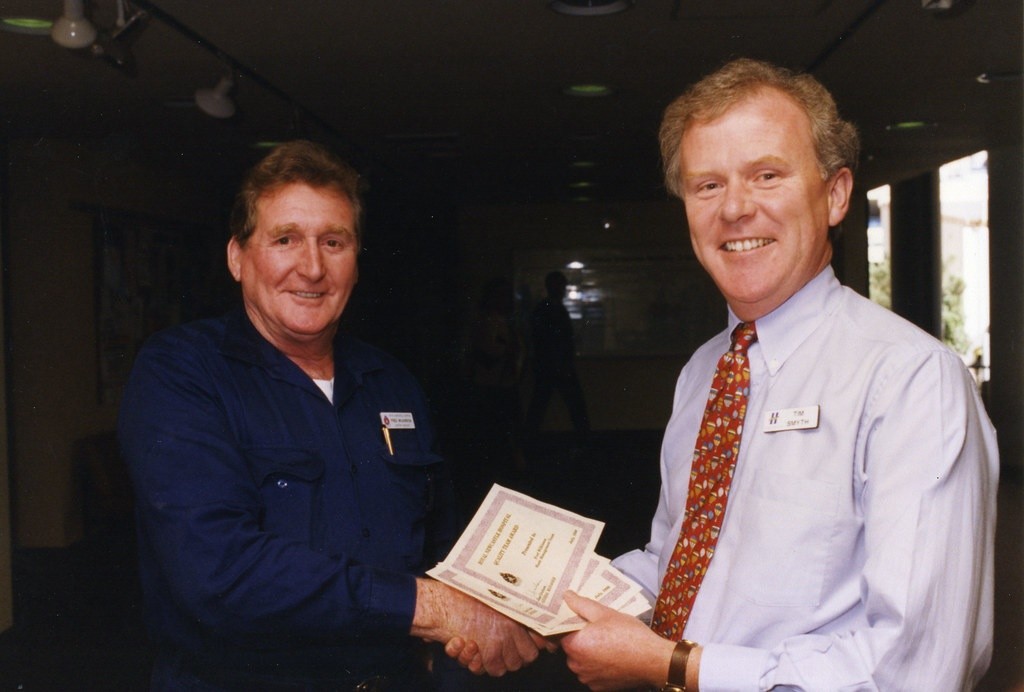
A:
381,422,398,461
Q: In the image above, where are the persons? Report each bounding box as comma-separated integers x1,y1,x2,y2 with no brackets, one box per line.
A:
120,136,547,691
445,60,1000,692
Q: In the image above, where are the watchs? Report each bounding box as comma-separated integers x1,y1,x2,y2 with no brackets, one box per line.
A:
661,640,698,692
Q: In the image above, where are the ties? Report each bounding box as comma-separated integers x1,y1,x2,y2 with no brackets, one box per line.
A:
649,320,757,643
